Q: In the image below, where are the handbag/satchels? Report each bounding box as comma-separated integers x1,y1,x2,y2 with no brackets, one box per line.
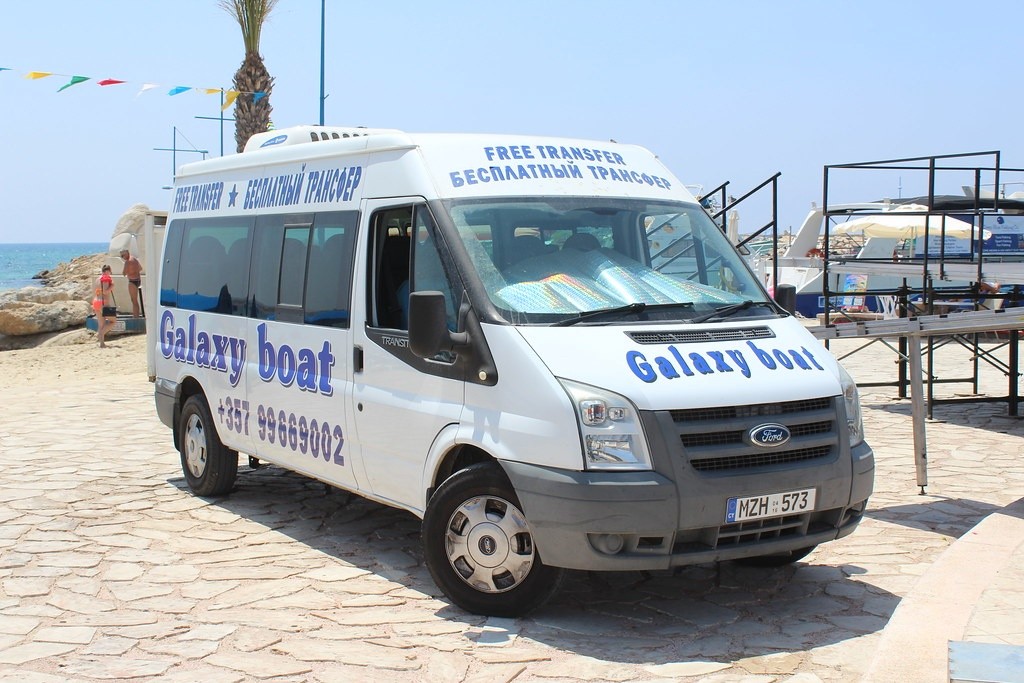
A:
101,306,117,318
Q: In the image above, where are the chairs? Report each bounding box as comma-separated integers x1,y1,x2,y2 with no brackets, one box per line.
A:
189,230,602,325
874,294,900,320
963,292,1008,343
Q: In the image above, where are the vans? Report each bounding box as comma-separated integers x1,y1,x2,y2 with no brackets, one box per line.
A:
142,124,876,621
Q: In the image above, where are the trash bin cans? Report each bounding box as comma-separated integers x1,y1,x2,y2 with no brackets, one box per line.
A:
777,284,796,316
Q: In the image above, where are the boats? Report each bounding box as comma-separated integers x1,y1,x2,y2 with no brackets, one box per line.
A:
642,180,1024,315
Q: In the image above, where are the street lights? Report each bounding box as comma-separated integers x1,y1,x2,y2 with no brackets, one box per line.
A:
153,125,210,184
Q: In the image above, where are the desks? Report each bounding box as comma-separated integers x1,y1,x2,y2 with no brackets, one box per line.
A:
912,299,979,340
816,312,885,325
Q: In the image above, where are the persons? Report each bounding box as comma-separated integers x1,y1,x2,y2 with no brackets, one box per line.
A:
119,249,143,320
93,265,116,349
